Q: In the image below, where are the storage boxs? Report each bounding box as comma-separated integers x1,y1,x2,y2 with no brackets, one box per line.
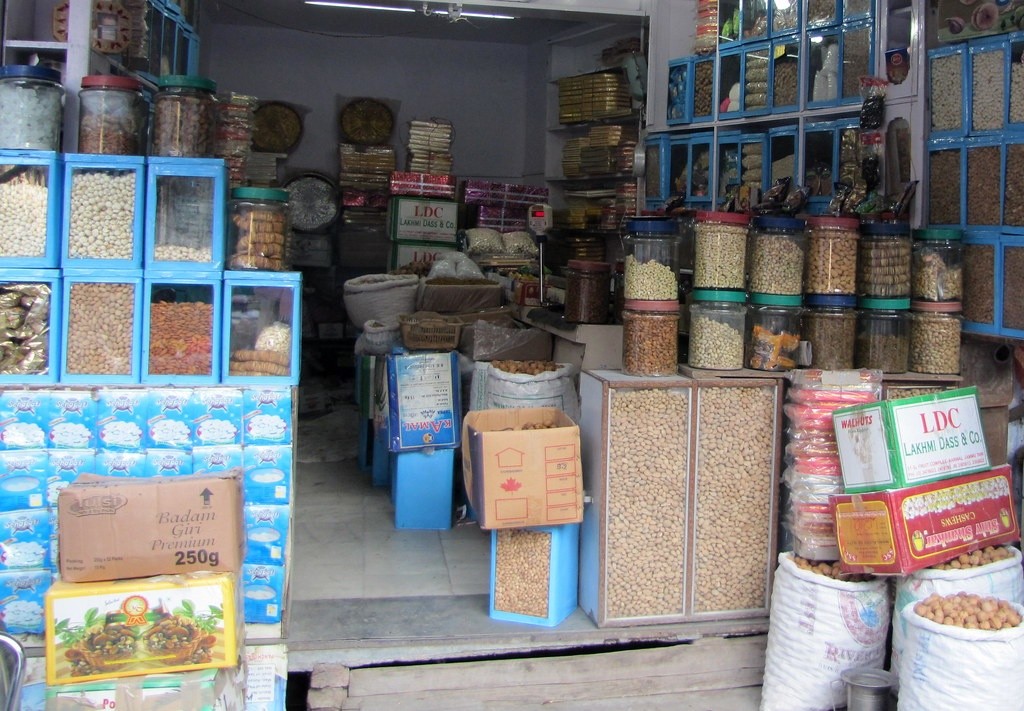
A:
388,197,461,270
46,663,246,711
832,385,990,494
419,278,552,362
46,573,244,686
385,350,461,454
644,0,875,214
1,149,302,387
147,0,201,86
461,407,584,530
58,473,244,582
927,0,1024,339
829,464,1020,576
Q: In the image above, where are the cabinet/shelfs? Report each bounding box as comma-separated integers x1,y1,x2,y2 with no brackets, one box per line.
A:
3,0,159,153
543,22,648,211
581,368,784,628
645,0,928,230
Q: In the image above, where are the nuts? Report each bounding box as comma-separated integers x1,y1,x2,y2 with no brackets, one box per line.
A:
789,557,875,582
608,385,774,617
0,171,290,378
624,46,1024,372
914,592,1022,632
923,546,1013,570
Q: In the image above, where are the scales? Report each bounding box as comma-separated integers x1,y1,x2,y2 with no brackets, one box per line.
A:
525,203,578,331
